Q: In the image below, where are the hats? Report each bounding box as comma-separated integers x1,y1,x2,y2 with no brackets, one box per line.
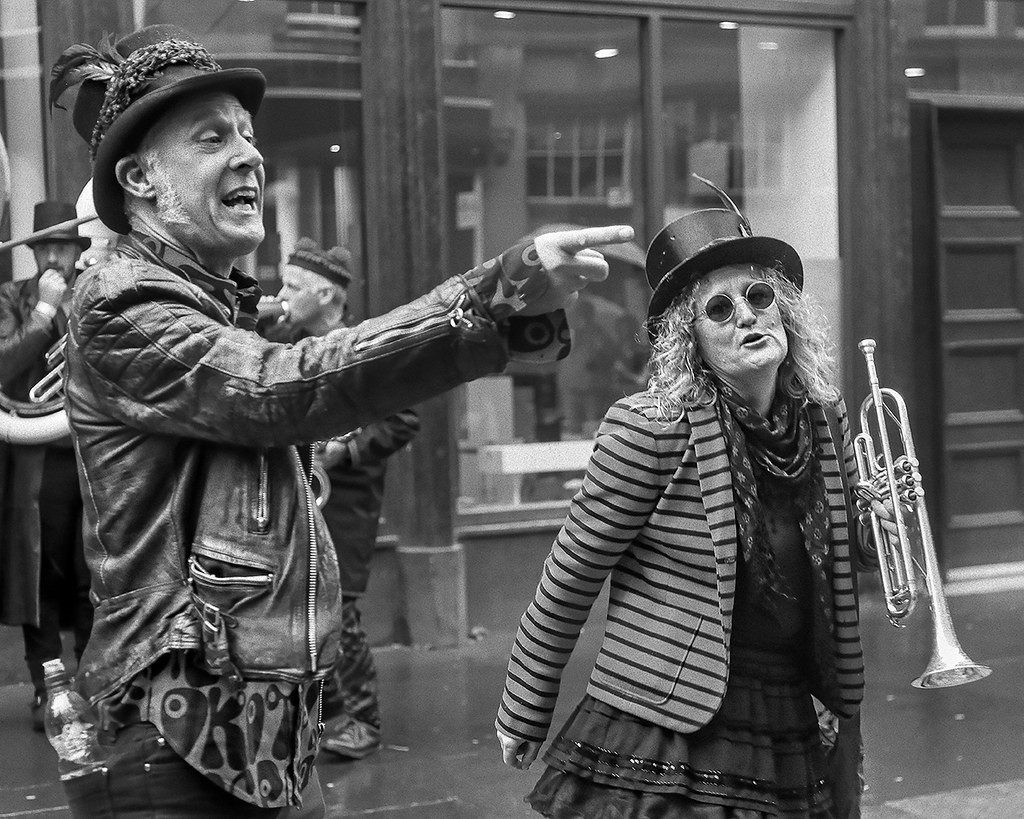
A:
24,200,92,252
288,239,351,289
646,173,804,344
49,24,266,238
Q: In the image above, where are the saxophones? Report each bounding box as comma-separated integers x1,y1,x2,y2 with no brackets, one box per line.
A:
310,426,362,515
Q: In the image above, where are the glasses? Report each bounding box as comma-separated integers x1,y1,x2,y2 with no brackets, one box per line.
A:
693,280,777,325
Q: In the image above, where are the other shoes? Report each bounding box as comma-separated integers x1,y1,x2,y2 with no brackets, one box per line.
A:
30,694,48,733
318,693,346,723
321,717,382,758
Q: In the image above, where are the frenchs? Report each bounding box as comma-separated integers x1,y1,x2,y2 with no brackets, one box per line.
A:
0,177,119,443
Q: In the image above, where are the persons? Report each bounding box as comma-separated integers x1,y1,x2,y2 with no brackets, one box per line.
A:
494,175,912,819
0,203,93,733
254,235,422,760
48,25,634,819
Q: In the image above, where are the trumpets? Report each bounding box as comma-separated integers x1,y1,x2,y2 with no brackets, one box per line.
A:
852,336,992,692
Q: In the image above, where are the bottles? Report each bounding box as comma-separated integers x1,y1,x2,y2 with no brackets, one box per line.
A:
42,657,103,773
256,301,288,326
